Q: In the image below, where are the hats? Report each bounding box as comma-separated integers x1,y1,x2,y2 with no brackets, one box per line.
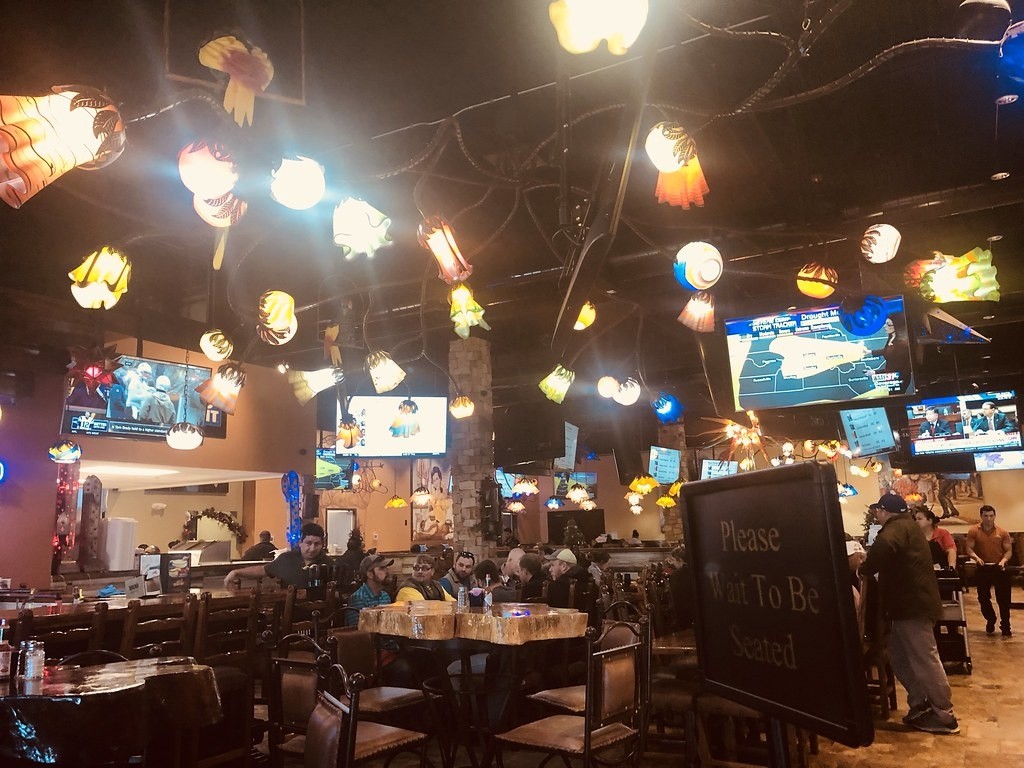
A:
870,494,909,513
358,555,394,577
544,548,577,565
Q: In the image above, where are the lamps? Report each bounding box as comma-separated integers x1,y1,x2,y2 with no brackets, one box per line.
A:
0,0,1024,514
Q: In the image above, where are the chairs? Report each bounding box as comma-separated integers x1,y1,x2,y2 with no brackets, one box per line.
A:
860,572,897,718
9,560,773,768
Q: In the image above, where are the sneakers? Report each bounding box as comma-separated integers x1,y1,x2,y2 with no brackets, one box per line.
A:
903,703,932,723
912,711,960,733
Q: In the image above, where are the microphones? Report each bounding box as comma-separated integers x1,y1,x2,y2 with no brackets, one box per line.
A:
974,415,986,431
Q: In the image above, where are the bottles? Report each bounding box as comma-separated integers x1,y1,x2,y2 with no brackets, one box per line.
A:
483,573,493,611
18,641,45,696
457,587,467,608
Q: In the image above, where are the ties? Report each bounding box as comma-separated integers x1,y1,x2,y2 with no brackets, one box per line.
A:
989,419,993,430
931,426,934,434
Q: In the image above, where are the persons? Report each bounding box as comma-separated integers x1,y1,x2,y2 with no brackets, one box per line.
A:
630,530,642,544
659,546,693,628
865,312,911,393
137,544,160,553
347,546,553,688
243,531,276,559
972,401,1013,433
502,528,519,546
105,363,177,423
958,409,976,433
856,494,960,732
222,523,338,599
543,549,601,630
919,407,950,435
916,511,957,570
966,506,1012,637
588,552,610,584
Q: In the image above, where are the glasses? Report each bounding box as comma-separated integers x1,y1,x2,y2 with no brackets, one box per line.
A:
461,552,473,557
413,567,431,572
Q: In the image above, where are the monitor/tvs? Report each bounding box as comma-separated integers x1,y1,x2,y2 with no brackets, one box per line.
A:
315,447,356,491
553,472,598,500
613,449,644,486
59,354,213,446
494,471,523,498
492,398,566,468
648,446,681,484
335,395,449,459
699,460,738,480
723,291,1024,474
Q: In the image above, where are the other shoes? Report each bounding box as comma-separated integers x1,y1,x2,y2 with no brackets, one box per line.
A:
986,617,996,633
949,510,959,516
1002,628,1012,636
939,513,950,519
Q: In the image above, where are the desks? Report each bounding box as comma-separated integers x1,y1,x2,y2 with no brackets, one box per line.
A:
357,600,589,768
0,589,308,649
0,653,221,768
